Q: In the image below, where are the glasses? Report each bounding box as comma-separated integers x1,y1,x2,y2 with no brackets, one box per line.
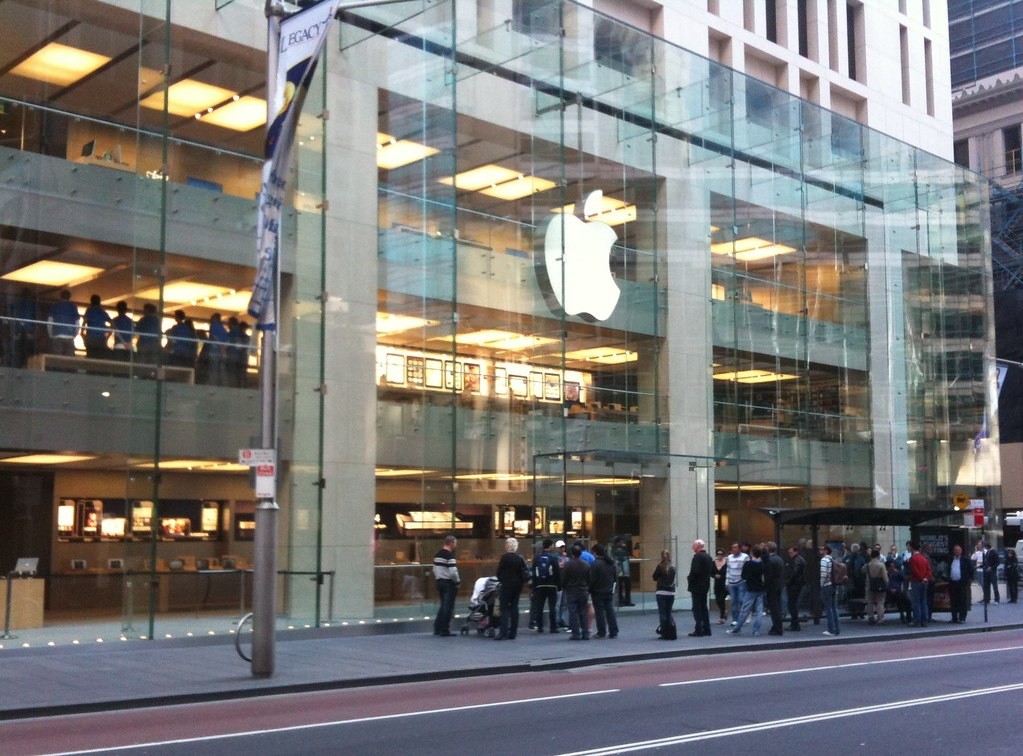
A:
717,553,724,556
820,548,824,551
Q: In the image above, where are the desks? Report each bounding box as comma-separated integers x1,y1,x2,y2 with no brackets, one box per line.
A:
719,423,798,439
0,578,45,631
564,409,639,424
27,353,195,385
72,155,130,171
50,559,503,608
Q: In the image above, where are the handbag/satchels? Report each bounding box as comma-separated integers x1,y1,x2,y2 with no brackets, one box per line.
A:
869,577,886,593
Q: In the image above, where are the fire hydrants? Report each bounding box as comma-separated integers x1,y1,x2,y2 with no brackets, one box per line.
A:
587,602,596,632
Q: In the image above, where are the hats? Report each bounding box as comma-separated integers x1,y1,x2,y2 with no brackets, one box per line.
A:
555,540,565,548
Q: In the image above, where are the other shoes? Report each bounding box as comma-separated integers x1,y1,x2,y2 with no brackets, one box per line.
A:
427,593,1019,664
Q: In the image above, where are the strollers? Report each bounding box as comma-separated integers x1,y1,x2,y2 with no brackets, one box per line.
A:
461,577,501,638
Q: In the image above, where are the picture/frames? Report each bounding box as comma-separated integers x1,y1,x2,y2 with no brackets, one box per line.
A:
384,353,580,402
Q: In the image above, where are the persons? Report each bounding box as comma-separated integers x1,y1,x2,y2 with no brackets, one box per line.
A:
948,546,974,623
495,537,526,640
1,287,251,388
526,534,633,640
651,550,678,640
714,537,935,636
971,541,999,606
433,535,461,637
686,539,713,636
1006,550,1020,603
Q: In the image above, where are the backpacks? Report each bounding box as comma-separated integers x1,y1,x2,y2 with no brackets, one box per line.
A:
832,559,848,586
533,554,554,582
